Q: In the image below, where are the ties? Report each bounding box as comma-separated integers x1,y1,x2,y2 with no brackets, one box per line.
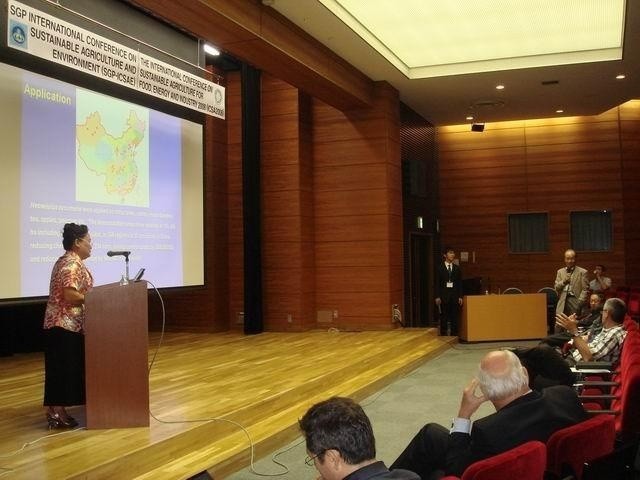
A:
448,265,451,278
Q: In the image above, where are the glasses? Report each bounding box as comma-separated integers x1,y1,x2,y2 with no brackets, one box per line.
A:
304,447,338,466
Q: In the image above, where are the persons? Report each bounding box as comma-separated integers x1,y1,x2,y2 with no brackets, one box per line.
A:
296,394,424,479
537,293,606,350
432,245,464,336
500,297,629,388
554,248,590,337
40,221,95,428
589,264,612,295
385,347,590,480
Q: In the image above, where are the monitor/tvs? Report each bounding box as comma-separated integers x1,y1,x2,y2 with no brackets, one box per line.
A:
133,268,145,282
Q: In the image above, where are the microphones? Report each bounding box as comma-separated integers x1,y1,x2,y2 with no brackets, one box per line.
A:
567,265,571,273
107,251,131,257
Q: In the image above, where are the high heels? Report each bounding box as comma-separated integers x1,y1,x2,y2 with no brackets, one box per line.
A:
45,409,79,428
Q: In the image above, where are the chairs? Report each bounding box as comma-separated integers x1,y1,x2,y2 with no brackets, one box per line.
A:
436,286,640,480
